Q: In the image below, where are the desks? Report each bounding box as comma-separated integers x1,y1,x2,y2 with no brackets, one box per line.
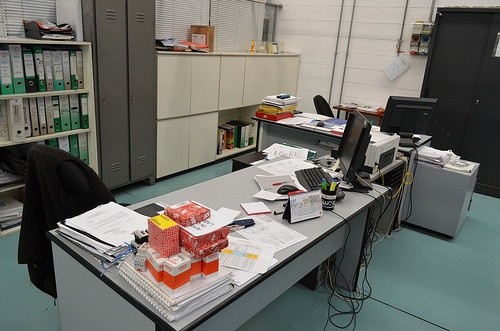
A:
45,102,433,331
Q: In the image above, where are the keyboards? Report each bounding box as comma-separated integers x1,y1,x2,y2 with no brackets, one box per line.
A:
295,168,345,200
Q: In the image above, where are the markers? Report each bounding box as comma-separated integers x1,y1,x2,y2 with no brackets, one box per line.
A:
322,178,326,190
230,223,255,232
329,179,338,191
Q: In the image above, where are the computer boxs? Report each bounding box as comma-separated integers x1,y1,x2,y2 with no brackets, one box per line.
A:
299,254,335,291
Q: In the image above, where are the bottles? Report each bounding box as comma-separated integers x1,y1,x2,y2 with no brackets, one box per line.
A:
272,41,278,54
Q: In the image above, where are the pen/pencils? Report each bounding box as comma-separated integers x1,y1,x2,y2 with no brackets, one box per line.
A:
283,202,288,207
227,219,254,227
336,180,343,187
274,211,284,215
272,180,295,185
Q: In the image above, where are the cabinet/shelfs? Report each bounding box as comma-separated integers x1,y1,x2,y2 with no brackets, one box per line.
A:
0,37,98,238
156,53,220,121
156,111,218,179
401,160,480,240
218,56,246,111
243,56,300,107
81,0,155,190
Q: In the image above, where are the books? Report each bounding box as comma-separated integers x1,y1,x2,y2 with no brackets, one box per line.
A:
118,260,234,322
217,120,254,155
0,194,23,231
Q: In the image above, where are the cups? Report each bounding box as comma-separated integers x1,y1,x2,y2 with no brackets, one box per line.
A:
320,188,337,211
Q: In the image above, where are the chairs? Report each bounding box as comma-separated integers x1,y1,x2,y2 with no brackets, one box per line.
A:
16,143,131,297
313,95,336,118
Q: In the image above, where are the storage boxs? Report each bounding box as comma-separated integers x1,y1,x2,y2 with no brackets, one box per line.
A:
147,199,231,295
190,24,215,53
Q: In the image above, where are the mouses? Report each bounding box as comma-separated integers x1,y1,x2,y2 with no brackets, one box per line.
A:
278,184,299,195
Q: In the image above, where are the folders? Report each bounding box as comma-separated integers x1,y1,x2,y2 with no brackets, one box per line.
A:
0,43,89,165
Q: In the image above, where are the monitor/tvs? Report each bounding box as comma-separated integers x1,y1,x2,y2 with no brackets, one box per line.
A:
337,109,373,194
382,96,437,133
